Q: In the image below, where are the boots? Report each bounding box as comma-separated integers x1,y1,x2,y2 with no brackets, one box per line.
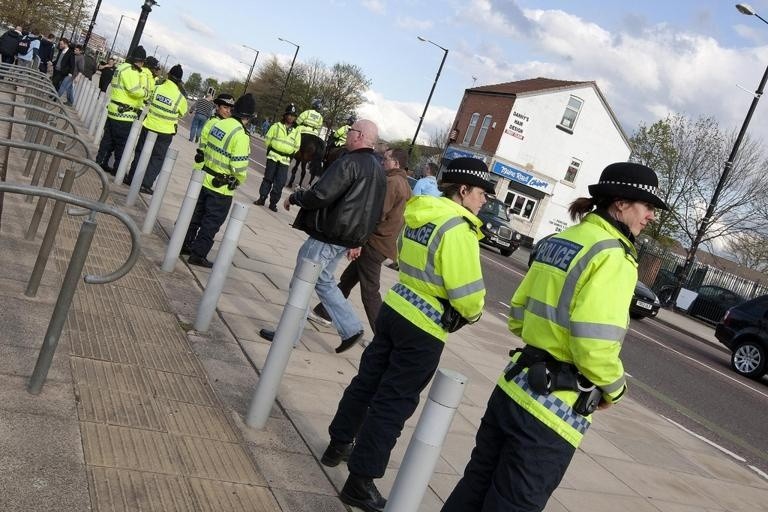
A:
254,196,266,206
321,438,357,467
269,199,279,212
340,471,388,511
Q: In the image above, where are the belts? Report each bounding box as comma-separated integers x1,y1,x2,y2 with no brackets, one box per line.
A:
271,147,291,157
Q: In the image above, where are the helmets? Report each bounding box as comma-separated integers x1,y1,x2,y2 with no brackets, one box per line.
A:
230,93,256,118
311,99,321,109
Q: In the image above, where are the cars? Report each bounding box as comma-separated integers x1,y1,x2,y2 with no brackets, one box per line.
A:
527,233,662,320
659,284,747,326
406,175,418,191
186,94,206,102
653,269,691,291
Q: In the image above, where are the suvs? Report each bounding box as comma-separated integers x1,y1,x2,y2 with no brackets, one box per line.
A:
713,293,768,380
476,193,523,258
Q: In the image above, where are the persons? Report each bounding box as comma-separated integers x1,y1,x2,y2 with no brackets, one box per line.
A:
83,48,98,82
405,167,423,181
296,98,324,138
257,118,388,353
1,26,85,105
95,46,188,195
320,155,500,512
305,146,414,339
179,92,256,270
254,102,301,211
384,161,444,272
330,116,355,147
246,112,272,139
188,93,213,143
441,161,670,512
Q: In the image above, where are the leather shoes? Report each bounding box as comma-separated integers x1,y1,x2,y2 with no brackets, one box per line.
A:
335,330,365,354
259,329,274,341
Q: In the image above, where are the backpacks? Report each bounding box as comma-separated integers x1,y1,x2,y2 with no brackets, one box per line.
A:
18,35,40,56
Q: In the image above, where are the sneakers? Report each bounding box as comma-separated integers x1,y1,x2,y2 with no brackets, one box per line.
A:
308,313,333,328
140,186,153,195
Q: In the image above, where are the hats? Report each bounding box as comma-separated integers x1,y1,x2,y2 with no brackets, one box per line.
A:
168,64,183,83
131,45,160,70
588,162,671,212
442,157,496,196
284,103,299,117
213,94,235,107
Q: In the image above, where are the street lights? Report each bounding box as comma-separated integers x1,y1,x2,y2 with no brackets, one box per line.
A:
106,15,137,59
409,34,449,165
271,37,300,122
242,45,260,96
126,0,160,63
153,44,165,58
240,62,253,96
160,54,171,80
142,32,153,39
667,3,768,313
